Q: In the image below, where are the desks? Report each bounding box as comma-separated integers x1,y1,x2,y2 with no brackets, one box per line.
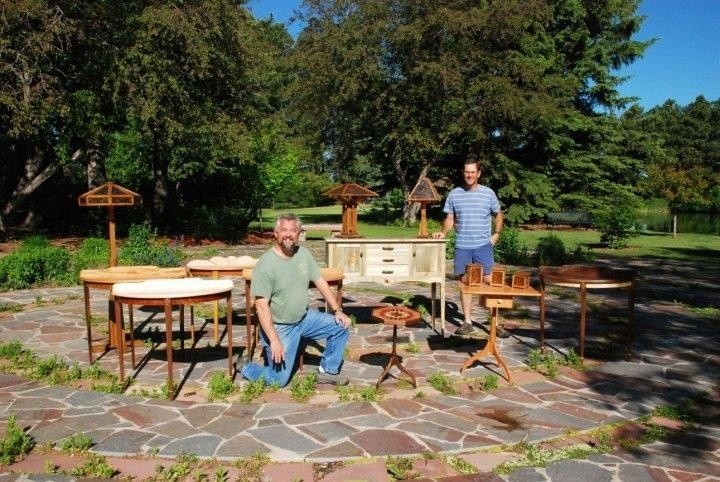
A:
371,308,421,394
81,265,187,366
109,277,236,397
239,267,344,362
186,260,257,346
540,265,640,366
457,282,541,382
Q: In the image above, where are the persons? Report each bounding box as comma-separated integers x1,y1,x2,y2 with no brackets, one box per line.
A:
234,214,352,388
433,155,511,338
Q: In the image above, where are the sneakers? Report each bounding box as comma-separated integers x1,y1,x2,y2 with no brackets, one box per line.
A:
455,320,474,335
315,369,349,386
496,325,510,338
232,355,250,372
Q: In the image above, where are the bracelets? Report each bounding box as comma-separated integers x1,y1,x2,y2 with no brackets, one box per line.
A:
333,308,343,313
495,232,501,235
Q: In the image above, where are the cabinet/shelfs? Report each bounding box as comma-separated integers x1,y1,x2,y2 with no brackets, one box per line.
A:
324,235,450,283
